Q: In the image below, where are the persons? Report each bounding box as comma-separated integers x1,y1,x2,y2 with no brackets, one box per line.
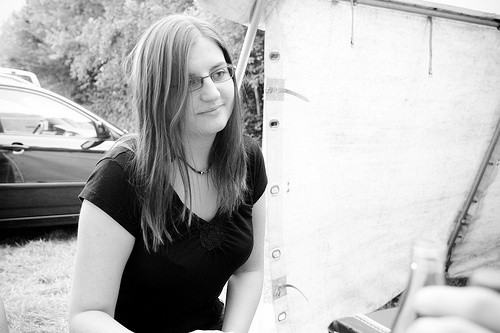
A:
67,13,270,333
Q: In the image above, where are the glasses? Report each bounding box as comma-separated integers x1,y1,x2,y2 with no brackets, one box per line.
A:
186,62,236,92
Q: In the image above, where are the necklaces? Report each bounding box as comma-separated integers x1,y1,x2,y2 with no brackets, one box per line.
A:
178,153,214,174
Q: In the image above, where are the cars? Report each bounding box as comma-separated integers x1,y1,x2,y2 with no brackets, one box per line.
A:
0,74,130,239
